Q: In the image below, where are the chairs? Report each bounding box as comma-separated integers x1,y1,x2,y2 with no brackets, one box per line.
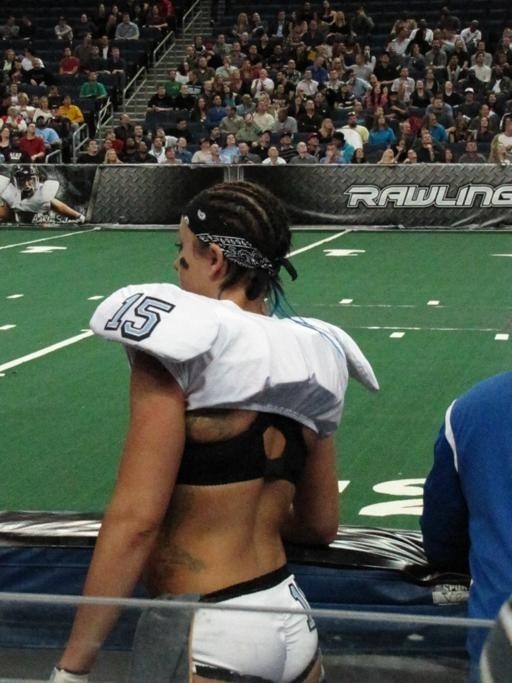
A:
211,0,512,161
0,0,189,132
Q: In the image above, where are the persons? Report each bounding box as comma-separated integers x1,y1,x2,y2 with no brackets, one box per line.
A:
49,181,380,683
73,0,511,197
0,164,85,223
2,1,179,186
418,370,512,683
